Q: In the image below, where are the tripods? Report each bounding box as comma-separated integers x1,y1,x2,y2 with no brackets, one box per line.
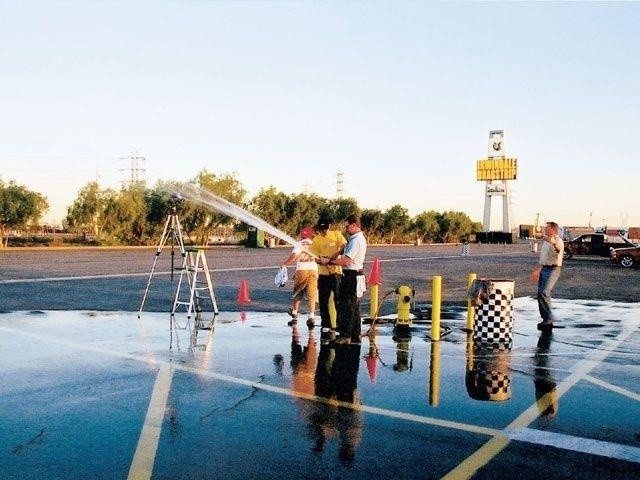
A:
138,213,202,319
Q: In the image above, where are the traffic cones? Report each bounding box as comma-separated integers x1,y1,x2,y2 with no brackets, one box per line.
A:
239,311,248,325
364,257,384,287
234,278,253,304
363,355,380,384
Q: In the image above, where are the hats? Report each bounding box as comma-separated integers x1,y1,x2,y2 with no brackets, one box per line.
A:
317,218,330,229
300,228,312,238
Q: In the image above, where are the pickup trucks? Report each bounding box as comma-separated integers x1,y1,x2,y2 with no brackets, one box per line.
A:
562,233,637,260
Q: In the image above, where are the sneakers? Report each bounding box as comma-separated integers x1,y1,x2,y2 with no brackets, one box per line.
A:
307,317,315,324
288,320,297,326
288,308,297,317
308,324,314,328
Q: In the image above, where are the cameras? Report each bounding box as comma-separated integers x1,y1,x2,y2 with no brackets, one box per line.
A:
168,193,185,206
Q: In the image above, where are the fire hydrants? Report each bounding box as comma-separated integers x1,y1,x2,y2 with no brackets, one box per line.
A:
392,330,416,373
394,284,419,333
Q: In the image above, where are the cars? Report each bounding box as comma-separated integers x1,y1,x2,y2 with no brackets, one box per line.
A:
609,231,640,269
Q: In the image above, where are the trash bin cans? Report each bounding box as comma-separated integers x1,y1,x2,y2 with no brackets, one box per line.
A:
268,238,276,249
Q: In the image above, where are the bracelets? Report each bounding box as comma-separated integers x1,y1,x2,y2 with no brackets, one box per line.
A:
328,258,336,265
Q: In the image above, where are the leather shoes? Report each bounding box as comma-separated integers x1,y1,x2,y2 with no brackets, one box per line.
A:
335,337,351,345
537,319,553,326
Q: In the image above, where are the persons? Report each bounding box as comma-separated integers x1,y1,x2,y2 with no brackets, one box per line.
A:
274,320,318,435
282,226,317,329
331,345,364,465
310,216,347,339
315,214,367,346
310,344,335,453
534,331,560,422
526,221,566,328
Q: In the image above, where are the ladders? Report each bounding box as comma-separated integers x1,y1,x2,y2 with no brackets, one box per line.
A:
170,248,219,319
